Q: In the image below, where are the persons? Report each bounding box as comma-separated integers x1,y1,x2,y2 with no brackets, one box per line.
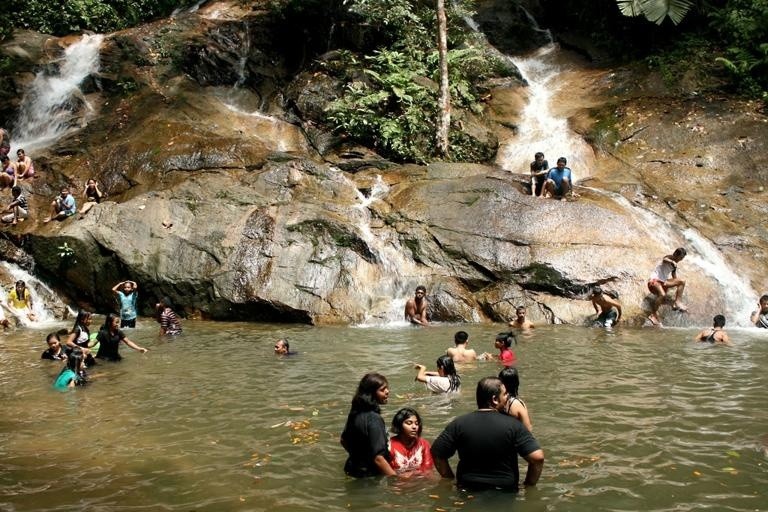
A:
694,315,735,346
404,285,432,328
530,152,549,199
750,295,768,329
275,338,303,361
647,247,688,325
41,280,184,395
589,288,622,327
545,157,572,202
7,280,38,322
0,127,103,225
